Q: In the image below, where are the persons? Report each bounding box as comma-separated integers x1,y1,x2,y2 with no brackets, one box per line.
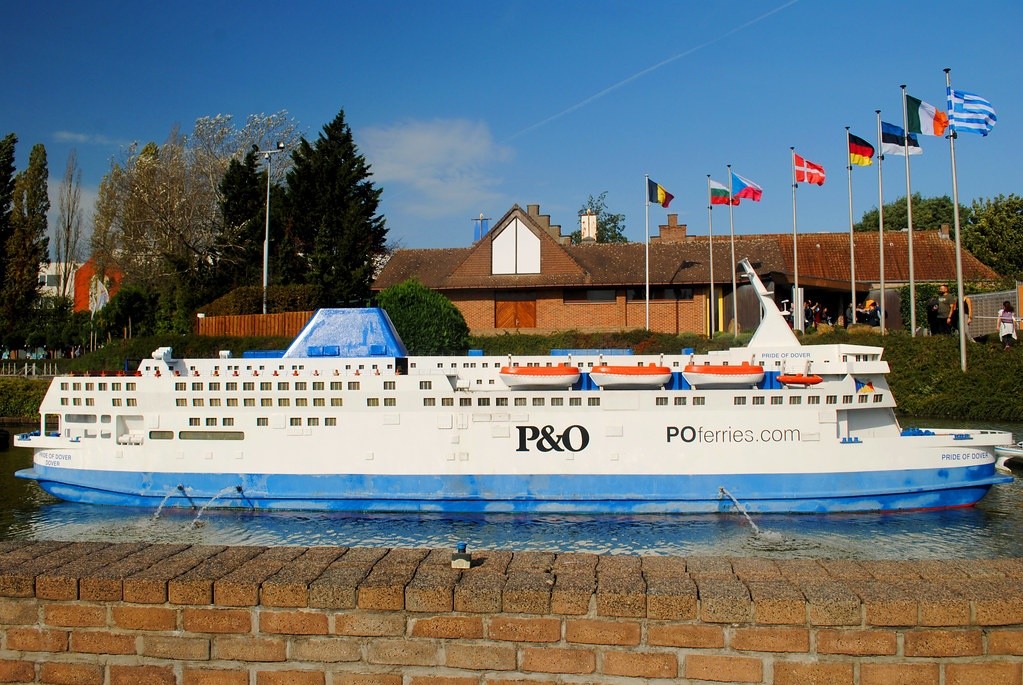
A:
932,286,977,344
846,300,888,327
781,300,833,328
996,300,1017,350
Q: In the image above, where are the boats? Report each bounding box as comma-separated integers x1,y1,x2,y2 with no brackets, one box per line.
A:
12,256,1023,515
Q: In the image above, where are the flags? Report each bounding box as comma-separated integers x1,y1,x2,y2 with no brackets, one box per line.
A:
648,178,675,208
731,171,763,202
710,180,740,206
906,94,949,136
794,152,826,186
880,121,923,155
947,86,998,137
849,133,875,166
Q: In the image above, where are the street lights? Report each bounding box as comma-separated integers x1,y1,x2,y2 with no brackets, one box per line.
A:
251,140,285,315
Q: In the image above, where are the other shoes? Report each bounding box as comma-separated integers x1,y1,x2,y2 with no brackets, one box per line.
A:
1004,344,1010,352
